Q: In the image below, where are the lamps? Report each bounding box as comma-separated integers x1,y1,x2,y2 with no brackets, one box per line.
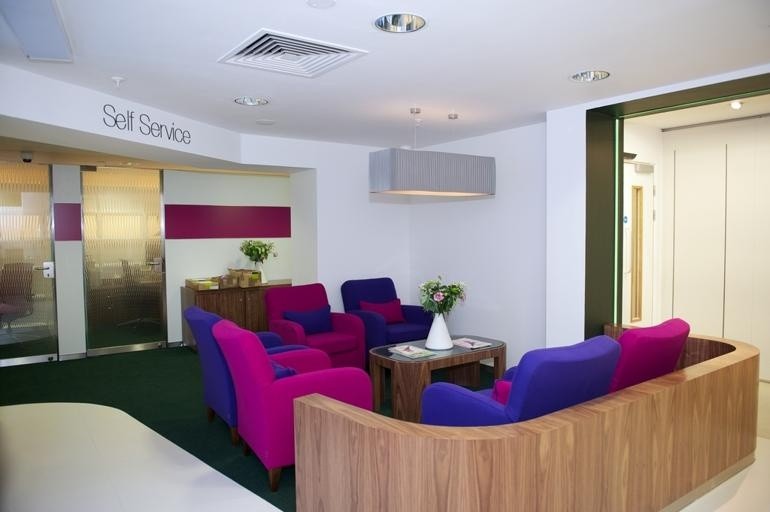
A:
365,107,498,201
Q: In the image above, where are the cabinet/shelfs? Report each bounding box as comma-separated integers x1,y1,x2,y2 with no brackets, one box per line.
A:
178,277,295,356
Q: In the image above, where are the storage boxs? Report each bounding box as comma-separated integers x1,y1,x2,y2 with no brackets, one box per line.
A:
227,267,263,289
184,278,220,293
208,275,241,291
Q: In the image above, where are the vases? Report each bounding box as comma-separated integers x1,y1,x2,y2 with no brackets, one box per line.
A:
252,260,269,285
422,310,455,352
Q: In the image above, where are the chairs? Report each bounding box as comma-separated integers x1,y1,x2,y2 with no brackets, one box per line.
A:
208,315,376,493
602,317,692,398
182,306,315,449
414,332,624,430
0,261,39,346
263,281,368,376
338,275,436,378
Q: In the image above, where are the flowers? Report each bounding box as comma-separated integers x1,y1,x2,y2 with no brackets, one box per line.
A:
238,239,279,266
412,271,467,322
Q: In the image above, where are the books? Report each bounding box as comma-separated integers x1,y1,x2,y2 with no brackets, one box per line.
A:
388,345,435,359
452,337,492,350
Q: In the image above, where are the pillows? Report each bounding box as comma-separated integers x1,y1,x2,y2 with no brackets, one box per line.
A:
489,377,514,406
270,360,298,382
357,295,406,324
281,302,334,337
